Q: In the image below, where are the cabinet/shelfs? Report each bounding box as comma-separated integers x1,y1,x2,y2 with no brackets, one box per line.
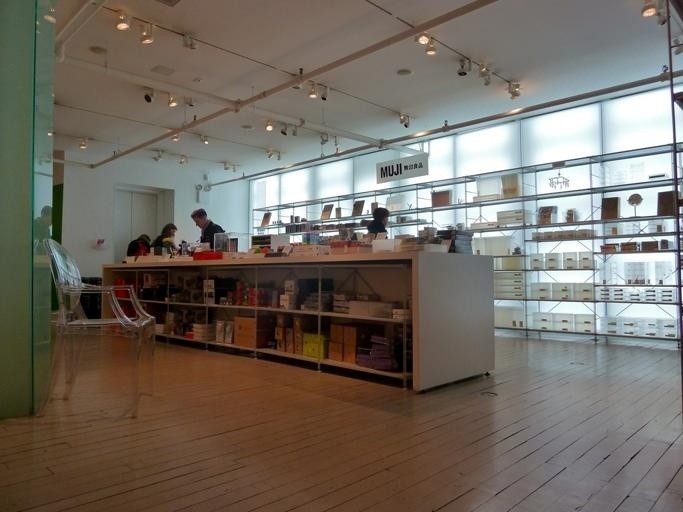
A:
100,251,493,390
467,141,683,349
253,174,475,243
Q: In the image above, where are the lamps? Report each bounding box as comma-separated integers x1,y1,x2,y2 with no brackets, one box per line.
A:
415,31,439,57
265,119,289,136
642,3,669,27
404,115,411,128
115,10,154,45
142,88,179,108
308,81,329,101
457,56,469,78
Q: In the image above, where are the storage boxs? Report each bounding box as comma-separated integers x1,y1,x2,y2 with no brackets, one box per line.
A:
234,315,367,364
432,189,452,207
602,196,620,219
658,191,681,216
202,279,238,304
284,280,334,308
346,299,392,318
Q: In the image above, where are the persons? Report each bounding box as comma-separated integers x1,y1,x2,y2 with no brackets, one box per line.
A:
191,208,225,252
176,240,189,255
126,234,151,261
150,224,177,256
367,208,390,240
32,205,52,255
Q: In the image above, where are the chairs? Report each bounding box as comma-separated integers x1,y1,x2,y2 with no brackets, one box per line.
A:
37,238,156,424
